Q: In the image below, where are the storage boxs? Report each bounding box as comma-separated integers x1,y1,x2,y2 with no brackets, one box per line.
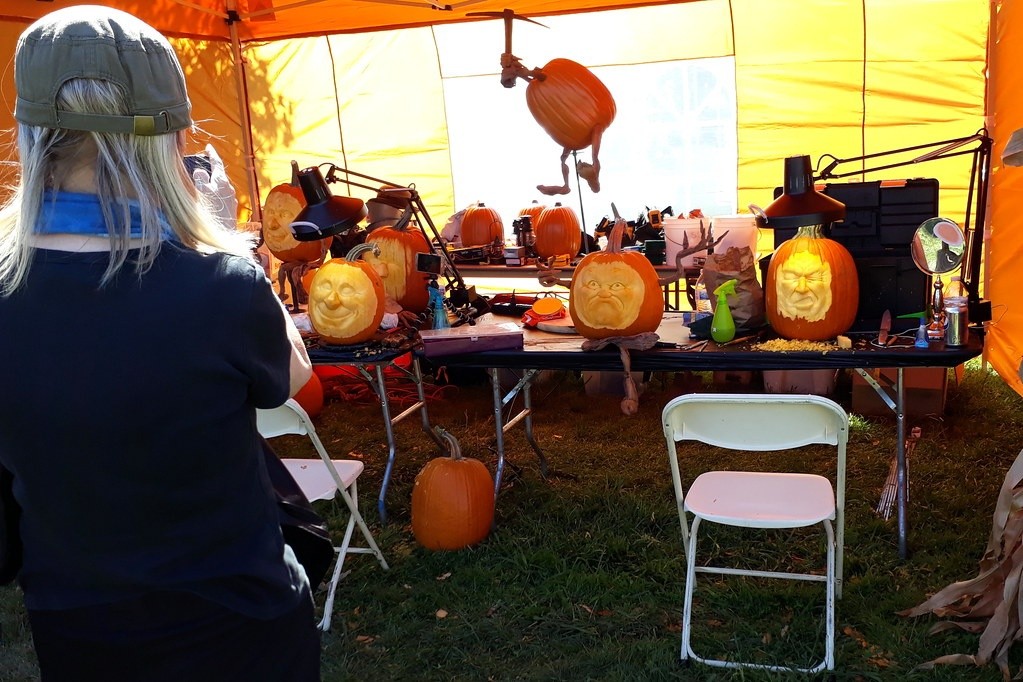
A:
712,371,763,387
583,371,654,398
763,369,837,400
853,362,966,419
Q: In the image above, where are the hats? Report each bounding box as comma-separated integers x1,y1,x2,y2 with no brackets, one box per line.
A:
13,2,194,134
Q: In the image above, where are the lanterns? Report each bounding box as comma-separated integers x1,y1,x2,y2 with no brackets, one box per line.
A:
259,160,334,263
766,226,860,341
569,219,666,343
308,204,437,346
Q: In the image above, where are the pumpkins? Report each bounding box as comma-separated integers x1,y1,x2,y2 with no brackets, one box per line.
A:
461,200,582,263
525,58,616,150
410,430,494,549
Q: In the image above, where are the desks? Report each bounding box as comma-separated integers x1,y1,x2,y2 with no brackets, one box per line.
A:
435,306,987,563
285,309,465,526
435,245,685,311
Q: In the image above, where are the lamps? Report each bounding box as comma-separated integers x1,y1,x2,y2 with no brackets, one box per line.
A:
754,128,995,323
1000,128,1023,168
291,162,482,308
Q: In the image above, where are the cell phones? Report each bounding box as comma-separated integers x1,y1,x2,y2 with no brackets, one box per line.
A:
182,154,211,185
416,251,444,275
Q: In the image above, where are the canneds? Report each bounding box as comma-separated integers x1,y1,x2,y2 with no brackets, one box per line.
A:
945,304,968,348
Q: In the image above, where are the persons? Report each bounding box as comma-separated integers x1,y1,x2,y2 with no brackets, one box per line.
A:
0,6,335,681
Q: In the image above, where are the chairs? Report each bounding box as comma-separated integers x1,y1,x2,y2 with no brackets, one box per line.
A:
661,392,849,674
255,398,390,632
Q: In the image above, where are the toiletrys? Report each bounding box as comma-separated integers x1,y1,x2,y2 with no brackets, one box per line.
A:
711,279,740,345
425,286,453,334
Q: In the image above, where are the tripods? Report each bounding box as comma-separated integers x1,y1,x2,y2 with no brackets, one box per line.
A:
406,276,476,338
